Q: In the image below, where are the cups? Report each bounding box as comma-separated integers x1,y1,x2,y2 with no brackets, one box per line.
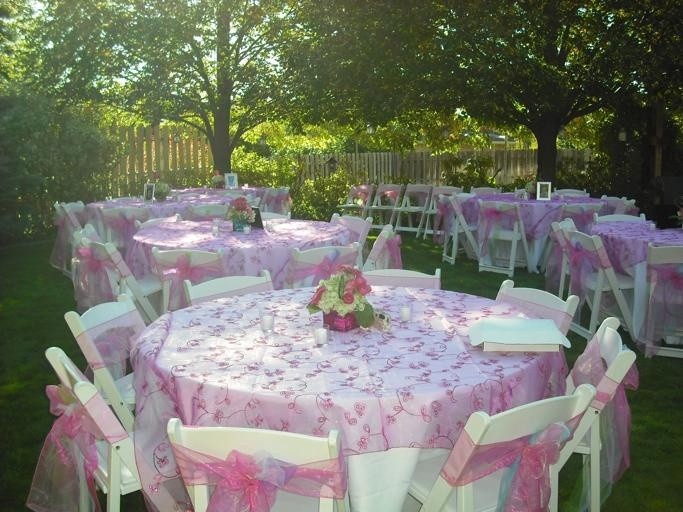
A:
258,310,274,334
314,324,329,345
244,182,248,188
211,218,220,238
242,223,251,235
399,306,410,322
